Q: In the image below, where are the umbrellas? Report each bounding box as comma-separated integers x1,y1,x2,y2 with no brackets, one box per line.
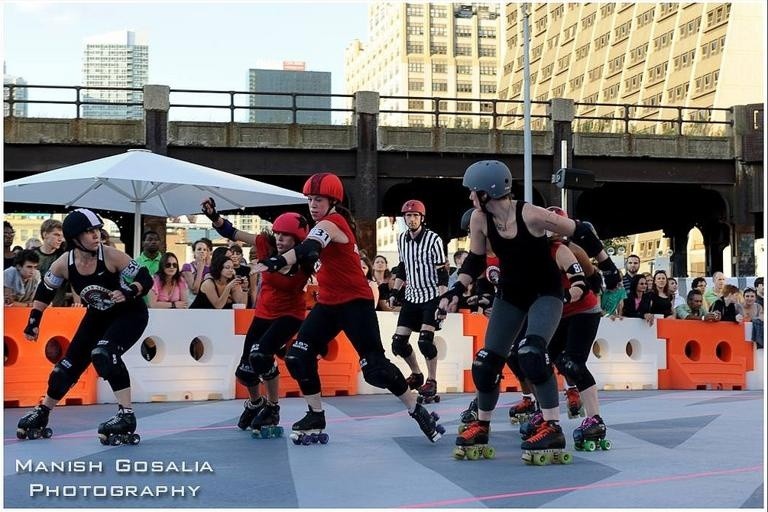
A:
3,148,310,261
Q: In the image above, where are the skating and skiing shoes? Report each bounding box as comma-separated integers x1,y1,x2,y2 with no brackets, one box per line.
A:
408,404,446,444
458,399,479,433
564,387,585,418
417,379,440,403
16,404,54,439
572,416,611,451
508,395,536,425
250,405,284,439
289,410,329,446
405,373,424,390
455,421,494,460
520,423,571,466
238,395,268,430
519,409,544,440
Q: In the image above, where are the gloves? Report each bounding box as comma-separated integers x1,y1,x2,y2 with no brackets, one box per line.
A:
440,281,468,306
203,202,221,222
435,306,447,317
256,253,288,274
596,256,622,290
24,308,43,337
116,284,139,301
385,287,400,306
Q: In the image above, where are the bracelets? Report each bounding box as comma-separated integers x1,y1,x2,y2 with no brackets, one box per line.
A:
701,316,705,321
171,301,176,308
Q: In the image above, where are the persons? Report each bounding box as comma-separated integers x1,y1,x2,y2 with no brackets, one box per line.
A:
378,267,404,311
386,200,450,405
640,270,676,327
249,172,446,443
34,219,84,308
212,246,232,257
448,250,468,290
666,278,685,307
197,196,310,438
180,240,210,308
642,272,654,290
597,278,627,318
754,277,764,308
624,274,648,318
230,245,256,309
676,289,721,322
121,231,162,303
227,238,247,265
622,254,640,292
25,237,42,250
3,250,42,308
508,205,610,426
742,287,762,322
358,257,379,309
704,272,725,305
200,238,213,267
691,277,708,312
434,160,623,466
2,218,16,270
458,208,537,433
149,252,188,309
217,294,219,295
11,246,23,256
709,284,743,323
189,256,249,310
372,254,388,286
544,236,612,453
16,209,153,447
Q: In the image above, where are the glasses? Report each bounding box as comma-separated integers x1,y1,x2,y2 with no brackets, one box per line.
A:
165,263,178,268
655,269,666,273
744,286,757,291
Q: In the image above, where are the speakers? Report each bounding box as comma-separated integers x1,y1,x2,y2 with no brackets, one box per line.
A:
554,167,597,191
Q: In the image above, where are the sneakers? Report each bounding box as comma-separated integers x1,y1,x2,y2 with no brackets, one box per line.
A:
97,410,141,446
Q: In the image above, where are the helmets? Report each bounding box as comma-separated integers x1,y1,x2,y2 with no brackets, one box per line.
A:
401,199,426,216
302,172,344,203
461,207,476,231
62,207,104,242
271,212,310,240
462,159,513,200
